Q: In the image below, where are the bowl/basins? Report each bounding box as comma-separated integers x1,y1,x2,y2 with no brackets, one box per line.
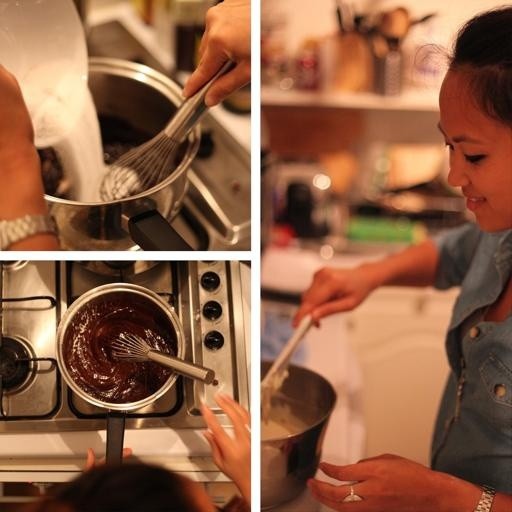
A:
261,360,339,512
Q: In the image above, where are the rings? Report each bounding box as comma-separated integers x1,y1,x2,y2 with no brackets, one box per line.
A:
341,486,363,503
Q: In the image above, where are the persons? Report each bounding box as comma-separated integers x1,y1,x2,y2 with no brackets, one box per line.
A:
0,1,250,251
285,4,512,512
25,390,251,510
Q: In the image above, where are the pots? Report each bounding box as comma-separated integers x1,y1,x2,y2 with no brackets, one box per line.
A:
55,280,188,465
30,57,203,251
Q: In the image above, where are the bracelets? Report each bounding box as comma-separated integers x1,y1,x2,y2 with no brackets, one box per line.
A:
0,213,61,250
471,482,496,512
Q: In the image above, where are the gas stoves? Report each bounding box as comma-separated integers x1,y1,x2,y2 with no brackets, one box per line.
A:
0,261,251,434
